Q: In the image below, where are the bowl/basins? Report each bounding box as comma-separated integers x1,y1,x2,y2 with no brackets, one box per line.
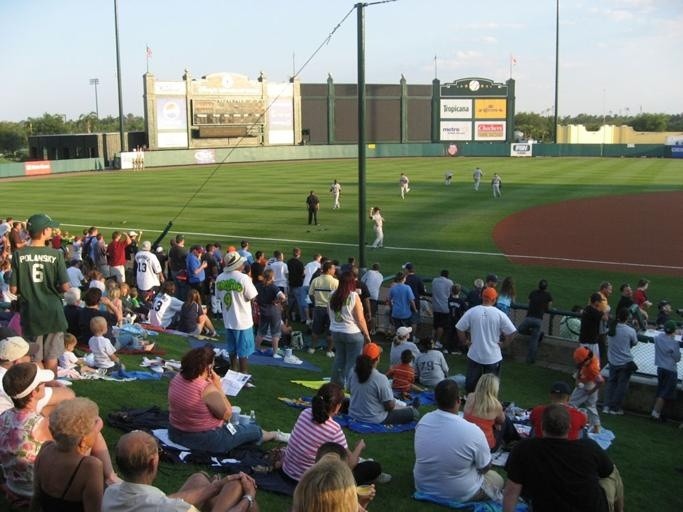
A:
137,341,156,351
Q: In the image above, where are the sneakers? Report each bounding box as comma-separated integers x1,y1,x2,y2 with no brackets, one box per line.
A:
372,472,392,483
326,351,335,358
308,347,316,354
276,428,291,442
283,355,302,365
602,406,610,413
609,409,624,415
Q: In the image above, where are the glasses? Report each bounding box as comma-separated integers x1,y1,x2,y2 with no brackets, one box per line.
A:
148,445,164,459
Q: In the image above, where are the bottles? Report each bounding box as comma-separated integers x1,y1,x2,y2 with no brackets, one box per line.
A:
249,410,255,425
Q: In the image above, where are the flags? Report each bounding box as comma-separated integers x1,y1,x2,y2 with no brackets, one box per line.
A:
146,46,152,58
511,55,519,67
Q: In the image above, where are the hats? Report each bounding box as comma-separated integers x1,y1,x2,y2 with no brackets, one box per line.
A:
1,336,39,362
363,342,383,360
572,348,589,364
8,361,56,398
401,262,414,270
141,241,151,251
473,274,501,300
26,213,61,233
222,250,247,273
396,326,412,337
663,317,677,333
129,231,138,236
550,379,572,396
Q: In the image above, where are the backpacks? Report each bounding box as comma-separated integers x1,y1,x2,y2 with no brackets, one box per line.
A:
81,236,93,260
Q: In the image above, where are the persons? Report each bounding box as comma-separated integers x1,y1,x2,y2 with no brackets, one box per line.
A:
491,173,502,199
412,269,682,511
445,170,454,185
399,172,411,200
472,167,483,192
306,190,319,225
1,214,419,509
369,208,385,247
330,179,342,208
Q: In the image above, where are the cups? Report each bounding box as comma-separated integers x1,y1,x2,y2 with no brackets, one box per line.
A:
229,406,241,425
358,485,372,495
238,415,250,424
284,349,292,359
